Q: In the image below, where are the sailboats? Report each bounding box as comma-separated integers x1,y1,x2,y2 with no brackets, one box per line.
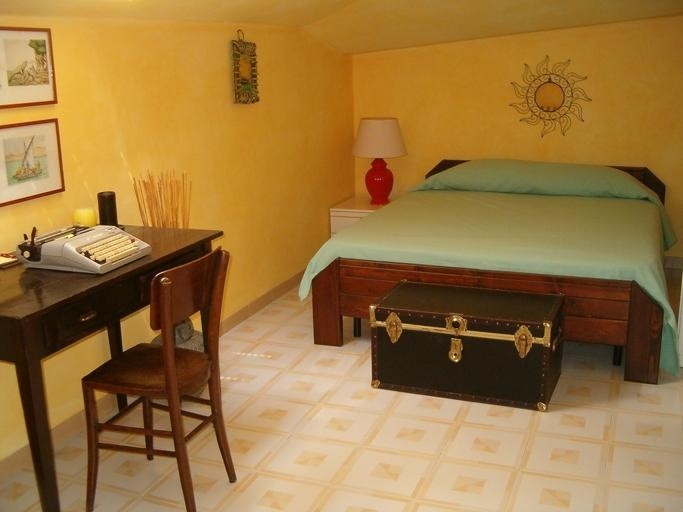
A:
10,136,43,181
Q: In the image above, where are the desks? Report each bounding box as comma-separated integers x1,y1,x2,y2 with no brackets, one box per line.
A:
0,225,223,512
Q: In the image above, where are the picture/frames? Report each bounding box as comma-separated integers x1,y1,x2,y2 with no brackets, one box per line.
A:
0,27,65,209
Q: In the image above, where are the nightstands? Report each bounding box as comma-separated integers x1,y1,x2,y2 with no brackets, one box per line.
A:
329,195,393,237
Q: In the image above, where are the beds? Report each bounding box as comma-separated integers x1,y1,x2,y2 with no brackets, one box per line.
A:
311,160,665,384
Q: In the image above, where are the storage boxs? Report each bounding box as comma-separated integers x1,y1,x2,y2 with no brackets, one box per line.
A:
368,280,566,412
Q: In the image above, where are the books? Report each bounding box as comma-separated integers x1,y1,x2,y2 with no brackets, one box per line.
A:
0,252,20,270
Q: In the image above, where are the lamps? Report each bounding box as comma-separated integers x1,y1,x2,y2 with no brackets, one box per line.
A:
352,118,408,204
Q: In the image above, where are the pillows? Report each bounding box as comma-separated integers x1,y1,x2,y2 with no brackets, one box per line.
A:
413,160,657,198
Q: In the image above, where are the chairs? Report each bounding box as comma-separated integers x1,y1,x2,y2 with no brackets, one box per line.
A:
81,246,236,512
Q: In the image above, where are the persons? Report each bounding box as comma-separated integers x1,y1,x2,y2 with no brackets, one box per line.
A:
7,53,47,83
